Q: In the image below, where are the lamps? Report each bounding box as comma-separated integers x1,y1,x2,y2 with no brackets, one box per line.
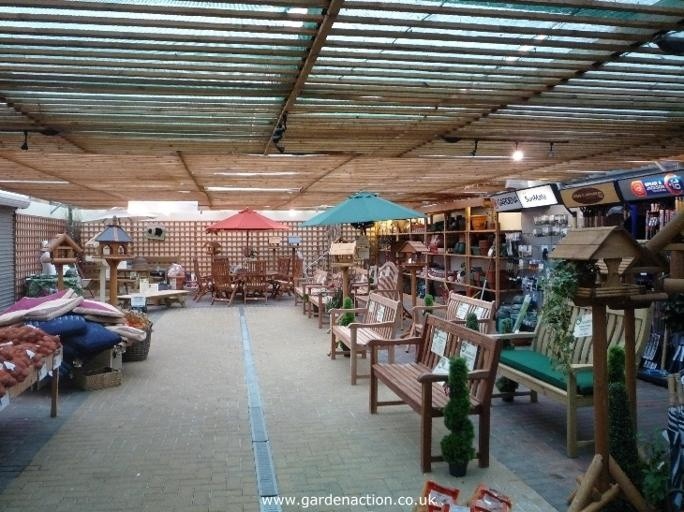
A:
547,142,556,161
511,139,524,162
20,132,28,150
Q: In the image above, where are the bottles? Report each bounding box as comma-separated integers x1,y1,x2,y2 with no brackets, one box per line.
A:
542,248,548,260
508,276,522,289
532,260,536,271
528,260,532,271
118,246,124,255
119,282,122,293
123,282,128,295
518,245,532,258
103,245,110,256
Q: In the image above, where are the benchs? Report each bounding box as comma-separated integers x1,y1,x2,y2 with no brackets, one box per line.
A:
485,303,653,458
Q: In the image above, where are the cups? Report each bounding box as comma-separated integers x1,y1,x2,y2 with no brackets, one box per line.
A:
139,279,158,295
130,272,139,280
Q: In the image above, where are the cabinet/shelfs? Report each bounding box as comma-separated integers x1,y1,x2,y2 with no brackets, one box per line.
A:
105,256,190,313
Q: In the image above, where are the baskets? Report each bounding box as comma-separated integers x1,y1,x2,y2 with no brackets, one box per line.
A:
123,321,153,361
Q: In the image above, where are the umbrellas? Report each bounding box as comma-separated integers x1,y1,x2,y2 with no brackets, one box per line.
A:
205,207,289,257
300,190,429,237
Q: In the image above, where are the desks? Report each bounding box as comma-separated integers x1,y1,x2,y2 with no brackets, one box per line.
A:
26,277,82,298
0,346,63,417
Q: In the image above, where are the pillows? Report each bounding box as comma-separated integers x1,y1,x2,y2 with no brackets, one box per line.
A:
24,312,122,380
